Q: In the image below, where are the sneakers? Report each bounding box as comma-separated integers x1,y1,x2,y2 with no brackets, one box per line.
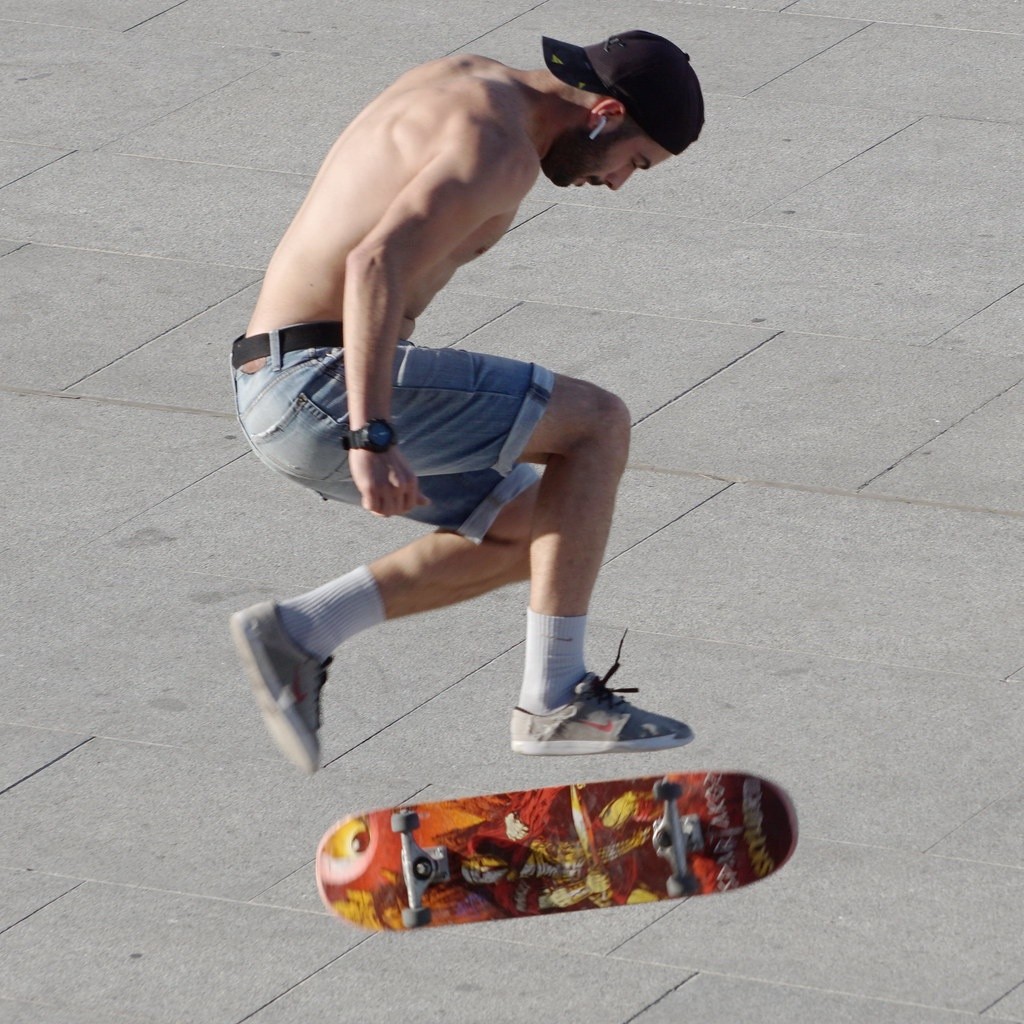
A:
230,598,335,772
510,629,694,756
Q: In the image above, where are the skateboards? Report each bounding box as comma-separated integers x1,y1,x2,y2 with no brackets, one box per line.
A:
316,769,800,934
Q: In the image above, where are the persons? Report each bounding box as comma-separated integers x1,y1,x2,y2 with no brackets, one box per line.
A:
232,32,705,770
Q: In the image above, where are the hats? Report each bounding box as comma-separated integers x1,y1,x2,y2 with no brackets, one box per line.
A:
541,30,706,157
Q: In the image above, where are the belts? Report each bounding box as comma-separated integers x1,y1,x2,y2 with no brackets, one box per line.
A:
231,320,343,370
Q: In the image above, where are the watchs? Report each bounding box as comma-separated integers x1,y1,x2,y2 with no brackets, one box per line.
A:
340,416,400,451
